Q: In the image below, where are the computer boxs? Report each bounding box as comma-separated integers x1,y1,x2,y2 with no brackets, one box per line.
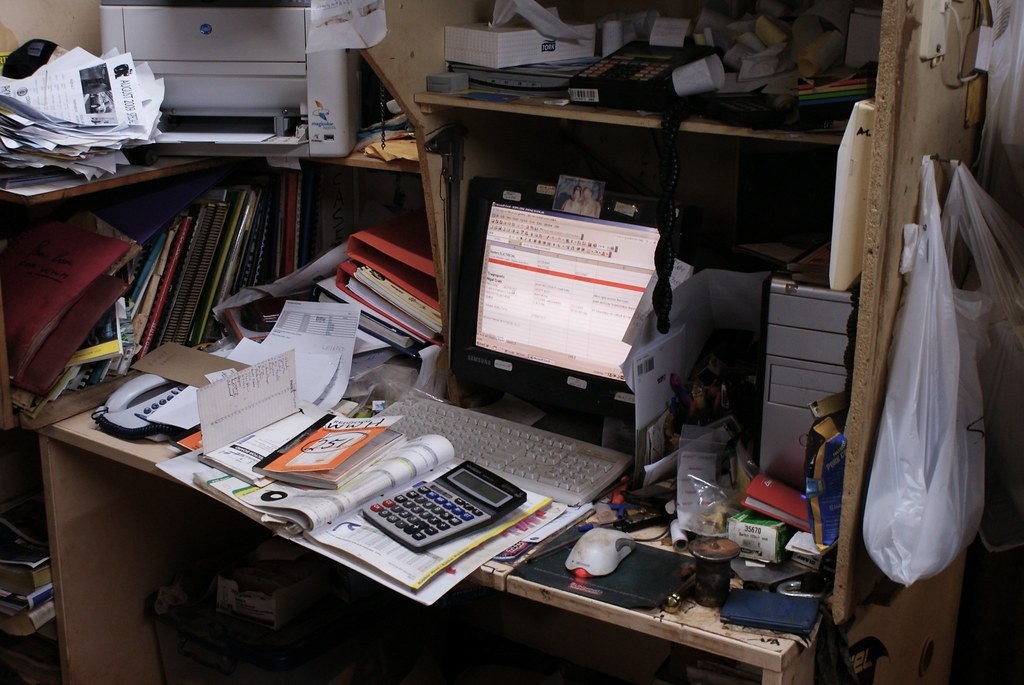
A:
756,235,857,499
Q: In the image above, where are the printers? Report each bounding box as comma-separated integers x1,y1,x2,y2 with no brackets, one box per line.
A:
99,0,360,160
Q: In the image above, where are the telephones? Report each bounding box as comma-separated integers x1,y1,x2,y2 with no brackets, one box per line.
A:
91,374,189,441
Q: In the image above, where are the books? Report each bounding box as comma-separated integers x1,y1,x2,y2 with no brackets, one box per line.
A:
719,590,819,635
742,474,811,532
0,182,552,636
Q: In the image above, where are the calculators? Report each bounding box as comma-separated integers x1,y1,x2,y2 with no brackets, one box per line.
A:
363,459,527,554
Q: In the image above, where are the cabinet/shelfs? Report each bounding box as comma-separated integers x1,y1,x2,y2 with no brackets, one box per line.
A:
0,159,421,430
359,0,981,623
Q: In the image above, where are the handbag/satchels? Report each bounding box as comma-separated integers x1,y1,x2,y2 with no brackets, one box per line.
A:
940,159,1024,554
862,155,993,588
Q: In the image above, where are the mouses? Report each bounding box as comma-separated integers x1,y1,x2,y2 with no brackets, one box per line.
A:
564,526,637,577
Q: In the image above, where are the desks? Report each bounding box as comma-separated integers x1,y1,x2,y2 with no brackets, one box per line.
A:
37,347,993,684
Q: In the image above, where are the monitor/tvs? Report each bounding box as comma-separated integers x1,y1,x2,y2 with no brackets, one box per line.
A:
450,174,699,446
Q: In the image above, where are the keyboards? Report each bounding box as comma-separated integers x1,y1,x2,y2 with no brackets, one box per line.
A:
371,395,633,507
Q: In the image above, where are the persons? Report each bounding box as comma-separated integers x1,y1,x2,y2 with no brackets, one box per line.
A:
561,186,602,220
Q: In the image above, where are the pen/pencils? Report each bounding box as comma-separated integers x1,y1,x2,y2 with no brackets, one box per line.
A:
668,372,739,428
578,519,627,531
